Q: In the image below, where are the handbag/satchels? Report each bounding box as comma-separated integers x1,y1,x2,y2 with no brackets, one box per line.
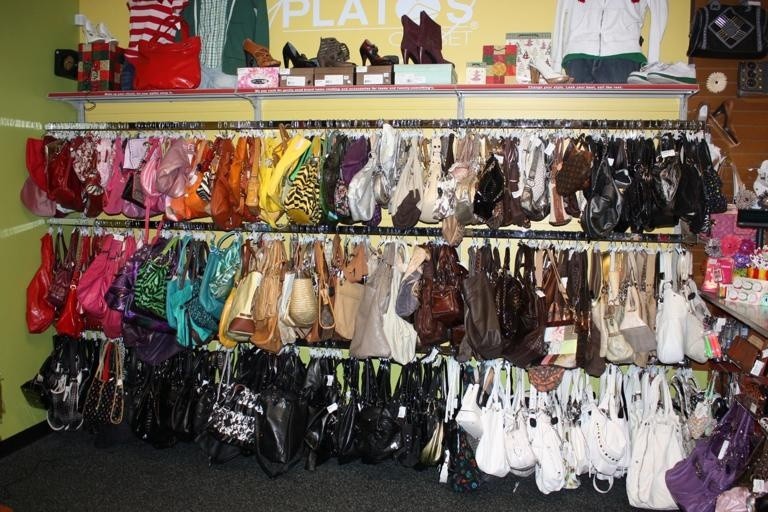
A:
20,123,728,235
19,336,752,512
24,230,720,368
132,15,201,90
702,204,756,293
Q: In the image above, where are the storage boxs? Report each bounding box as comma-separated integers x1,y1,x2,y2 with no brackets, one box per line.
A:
238,62,457,87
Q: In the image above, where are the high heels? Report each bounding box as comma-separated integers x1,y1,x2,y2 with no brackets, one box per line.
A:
360,39,392,66
529,55,574,85
82,21,119,43
283,37,356,68
398,11,456,67
243,39,281,67
712,100,740,144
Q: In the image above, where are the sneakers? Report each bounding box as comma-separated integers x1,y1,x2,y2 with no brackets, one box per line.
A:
627,61,696,85
